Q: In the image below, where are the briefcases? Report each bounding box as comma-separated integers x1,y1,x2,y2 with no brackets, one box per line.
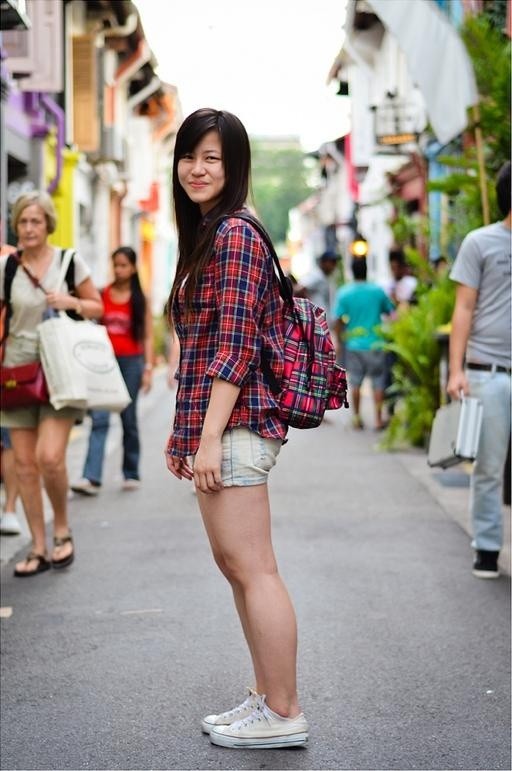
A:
424,388,484,471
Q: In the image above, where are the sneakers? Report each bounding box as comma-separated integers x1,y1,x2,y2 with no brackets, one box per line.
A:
121,477,142,491
0,510,21,536
200,685,263,735
209,694,310,751
471,548,501,580
69,476,100,497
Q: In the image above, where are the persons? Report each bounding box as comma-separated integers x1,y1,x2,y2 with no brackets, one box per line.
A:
307,252,342,352
163,108,309,752
388,249,419,304
66,247,155,497
432,256,447,273
1,192,103,575
329,256,395,433
0,426,24,536
445,162,510,581
382,274,401,309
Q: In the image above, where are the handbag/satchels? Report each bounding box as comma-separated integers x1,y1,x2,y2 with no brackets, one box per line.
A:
34,315,135,415
0,359,50,413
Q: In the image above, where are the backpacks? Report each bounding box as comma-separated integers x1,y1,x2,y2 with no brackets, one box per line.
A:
276,284,351,431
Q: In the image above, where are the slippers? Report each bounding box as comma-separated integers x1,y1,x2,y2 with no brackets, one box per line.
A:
14,551,53,577
51,526,75,571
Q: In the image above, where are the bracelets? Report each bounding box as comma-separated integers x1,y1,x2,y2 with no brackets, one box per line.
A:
144,365,153,371
75,298,82,315
